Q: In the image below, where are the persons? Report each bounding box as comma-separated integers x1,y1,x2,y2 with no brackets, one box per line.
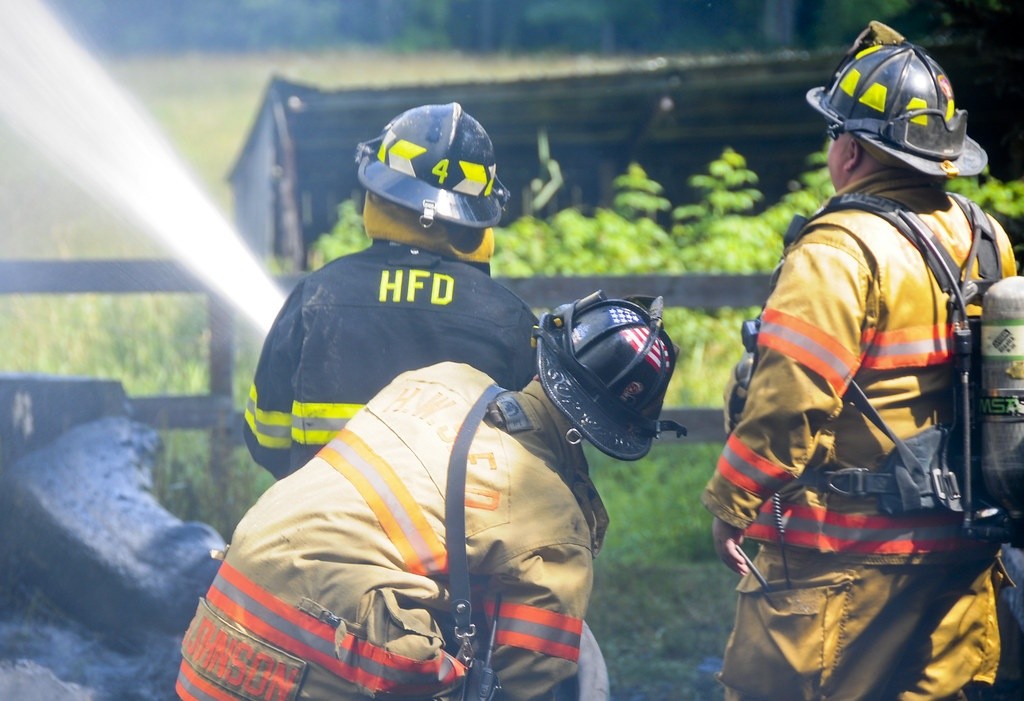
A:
244,103,539,483
701,24,1024,701
175,290,676,701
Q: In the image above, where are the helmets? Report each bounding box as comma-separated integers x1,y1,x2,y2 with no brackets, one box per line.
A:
806,20,988,179
530,290,688,461
355,102,511,229
